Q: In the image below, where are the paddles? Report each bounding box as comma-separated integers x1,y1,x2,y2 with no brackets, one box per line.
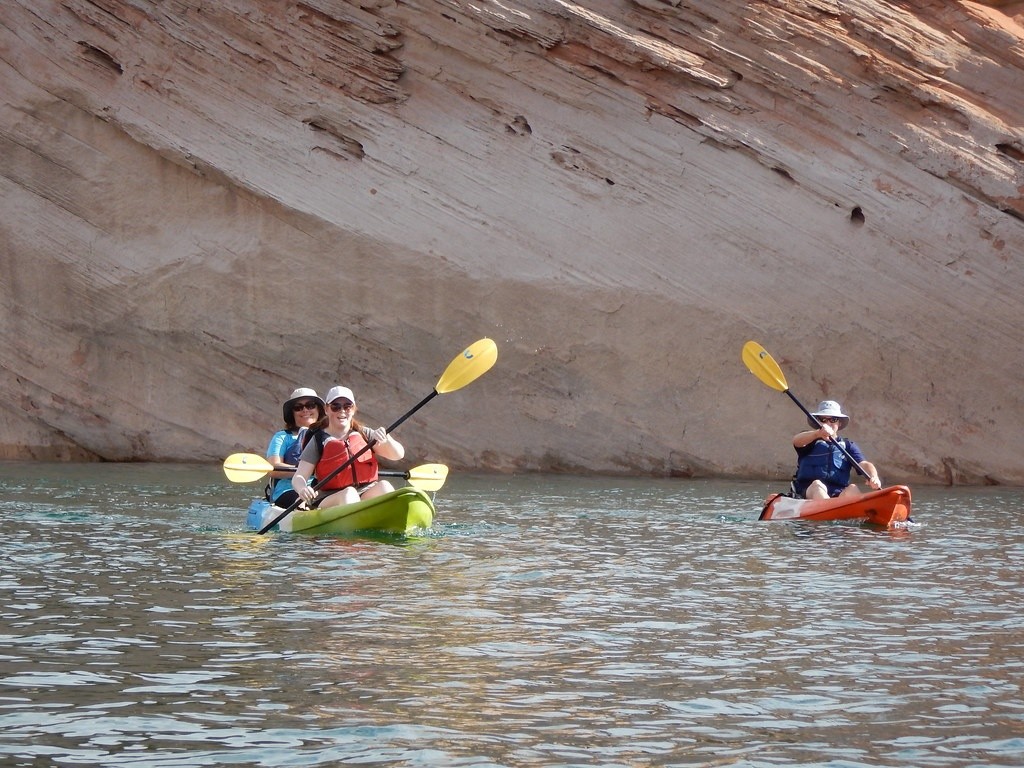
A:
223,452,450,492
741,341,915,523
254,337,499,535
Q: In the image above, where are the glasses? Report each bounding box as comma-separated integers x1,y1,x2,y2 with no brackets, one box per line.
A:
815,416,840,423
290,400,316,412
328,402,353,413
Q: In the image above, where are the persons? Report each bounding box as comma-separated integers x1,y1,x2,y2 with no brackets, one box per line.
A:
792,400,881,500
291,386,405,510
267,388,327,510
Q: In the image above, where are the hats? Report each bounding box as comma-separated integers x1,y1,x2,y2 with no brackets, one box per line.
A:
282,388,326,426
326,386,355,407
808,401,849,431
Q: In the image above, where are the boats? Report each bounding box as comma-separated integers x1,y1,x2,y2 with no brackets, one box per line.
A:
243,490,442,540
756,484,914,535
